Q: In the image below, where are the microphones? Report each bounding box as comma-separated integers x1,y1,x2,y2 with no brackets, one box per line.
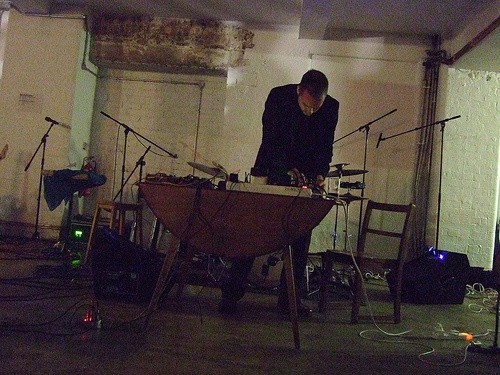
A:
172,154,179,158
45,117,59,125
376,133,383,149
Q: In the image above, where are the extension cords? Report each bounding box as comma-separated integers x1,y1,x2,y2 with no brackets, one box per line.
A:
225,180,312,197
431,331,472,341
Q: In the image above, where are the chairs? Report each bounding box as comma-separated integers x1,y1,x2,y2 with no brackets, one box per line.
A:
318,200,413,324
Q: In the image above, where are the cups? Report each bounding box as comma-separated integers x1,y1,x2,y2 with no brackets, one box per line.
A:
327,176,340,200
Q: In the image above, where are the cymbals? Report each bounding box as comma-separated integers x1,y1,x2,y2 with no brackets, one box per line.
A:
186,160,228,180
325,169,368,177
338,193,369,202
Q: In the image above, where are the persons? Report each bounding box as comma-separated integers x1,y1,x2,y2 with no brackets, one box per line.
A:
218,69,340,317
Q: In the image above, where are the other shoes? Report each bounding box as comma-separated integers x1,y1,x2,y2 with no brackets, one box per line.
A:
219,298,237,313
276,301,313,316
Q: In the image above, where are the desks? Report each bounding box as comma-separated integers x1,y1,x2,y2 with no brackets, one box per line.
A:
136,179,336,349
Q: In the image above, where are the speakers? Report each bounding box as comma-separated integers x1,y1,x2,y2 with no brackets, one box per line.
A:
386,250,469,304
85,226,159,297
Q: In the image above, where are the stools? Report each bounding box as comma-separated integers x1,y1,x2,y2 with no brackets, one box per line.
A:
84,202,142,265
